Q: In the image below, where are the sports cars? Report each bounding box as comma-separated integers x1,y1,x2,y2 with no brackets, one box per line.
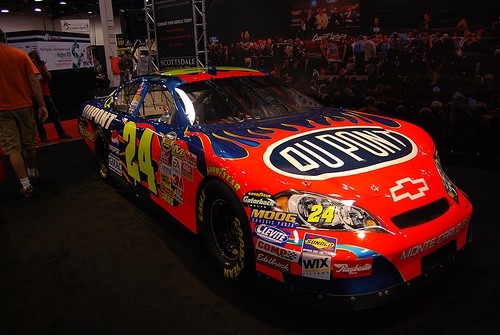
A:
77,66,480,313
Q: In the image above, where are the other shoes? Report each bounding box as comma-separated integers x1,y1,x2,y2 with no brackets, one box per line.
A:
28,175,40,185
18,185,33,198
59,134,71,138
41,137,48,142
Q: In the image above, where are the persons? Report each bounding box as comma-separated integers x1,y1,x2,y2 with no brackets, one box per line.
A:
27,50,72,142
94,13,500,152
0,29,49,196
